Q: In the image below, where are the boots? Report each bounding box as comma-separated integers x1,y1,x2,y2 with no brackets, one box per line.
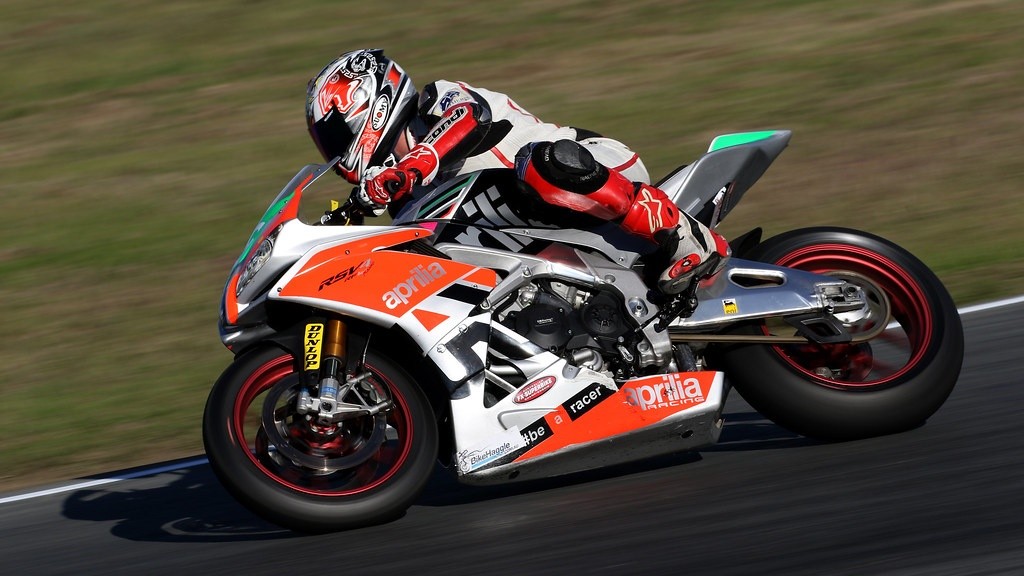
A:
620,180,732,295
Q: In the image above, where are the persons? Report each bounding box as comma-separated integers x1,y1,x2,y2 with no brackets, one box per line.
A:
305,48,732,293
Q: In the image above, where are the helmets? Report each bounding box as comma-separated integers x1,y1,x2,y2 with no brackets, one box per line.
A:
304,48,420,186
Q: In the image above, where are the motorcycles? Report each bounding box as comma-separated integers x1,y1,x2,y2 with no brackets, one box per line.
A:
202,130,964,524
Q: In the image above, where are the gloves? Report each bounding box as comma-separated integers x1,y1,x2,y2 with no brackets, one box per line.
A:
357,142,440,218
